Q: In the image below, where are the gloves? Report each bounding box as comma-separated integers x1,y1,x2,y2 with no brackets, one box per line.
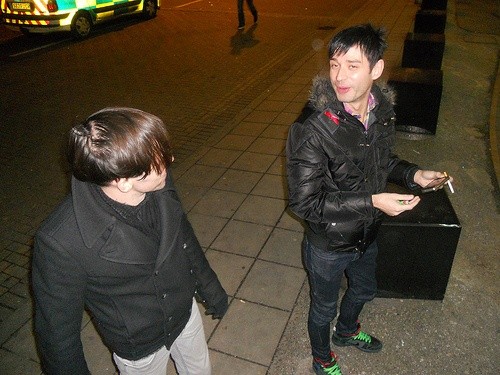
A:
206,293,229,319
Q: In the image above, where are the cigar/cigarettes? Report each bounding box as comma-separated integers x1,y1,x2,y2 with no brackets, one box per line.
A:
444,171,454,193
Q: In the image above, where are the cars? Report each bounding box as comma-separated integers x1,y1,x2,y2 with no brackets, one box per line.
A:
0,0,162,40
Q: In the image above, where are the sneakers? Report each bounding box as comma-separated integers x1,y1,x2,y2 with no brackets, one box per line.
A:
332,322,382,353
312,351,343,375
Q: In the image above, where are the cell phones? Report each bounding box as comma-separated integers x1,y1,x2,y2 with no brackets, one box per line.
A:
422,176,450,192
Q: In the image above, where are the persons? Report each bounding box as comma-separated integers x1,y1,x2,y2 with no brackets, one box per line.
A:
237,0,258,30
31,107,228,375
285,23,454,375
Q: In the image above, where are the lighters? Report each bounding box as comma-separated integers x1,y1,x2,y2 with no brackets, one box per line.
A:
397,200,411,205
